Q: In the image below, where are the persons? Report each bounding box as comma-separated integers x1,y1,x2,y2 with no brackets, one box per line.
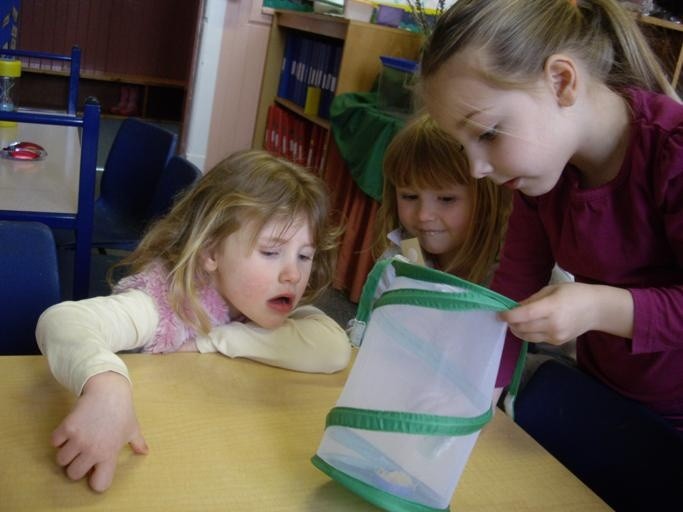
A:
35,147,351,492
376,112,516,290
418,0,683,512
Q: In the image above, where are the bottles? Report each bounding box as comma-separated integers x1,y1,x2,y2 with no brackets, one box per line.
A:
0,59,22,128
374,53,417,113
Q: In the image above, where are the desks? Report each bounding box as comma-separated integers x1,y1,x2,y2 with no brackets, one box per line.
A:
0,342,616,512
0,45,101,299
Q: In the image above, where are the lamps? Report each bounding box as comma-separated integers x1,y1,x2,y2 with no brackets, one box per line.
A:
0,60,21,112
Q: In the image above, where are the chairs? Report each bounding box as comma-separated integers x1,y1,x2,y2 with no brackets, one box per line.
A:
60,156,202,300
0,221,60,356
55,117,180,245
514,360,683,512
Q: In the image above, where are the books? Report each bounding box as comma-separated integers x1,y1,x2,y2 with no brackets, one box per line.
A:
261,29,388,304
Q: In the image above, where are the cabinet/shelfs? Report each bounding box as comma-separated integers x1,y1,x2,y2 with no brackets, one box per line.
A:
250,10,420,181
22,66,187,125
629,11,683,101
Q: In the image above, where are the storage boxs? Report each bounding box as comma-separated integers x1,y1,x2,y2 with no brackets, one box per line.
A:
378,55,419,116
